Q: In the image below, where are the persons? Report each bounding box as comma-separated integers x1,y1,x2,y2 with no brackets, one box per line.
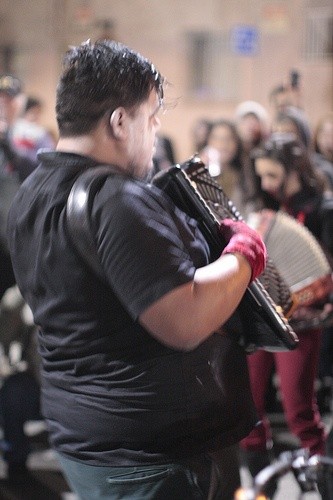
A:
0,69,333,500
7,40,269,500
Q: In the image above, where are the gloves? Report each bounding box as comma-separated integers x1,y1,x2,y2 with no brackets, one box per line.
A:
219,219,267,283
